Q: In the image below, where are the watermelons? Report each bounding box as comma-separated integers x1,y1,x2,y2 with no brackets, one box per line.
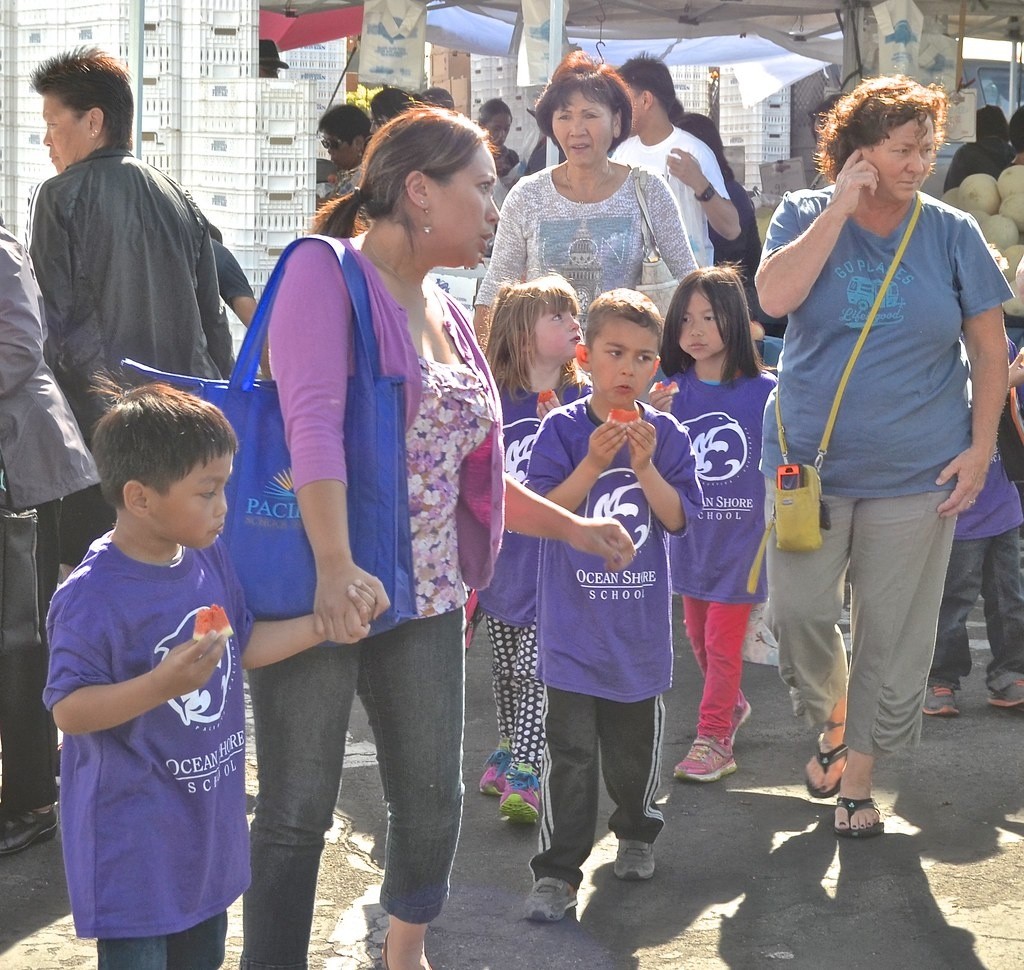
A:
607,408,641,427
538,389,559,402
192,604,234,640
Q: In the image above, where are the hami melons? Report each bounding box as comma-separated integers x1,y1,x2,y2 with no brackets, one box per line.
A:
935,165,1024,317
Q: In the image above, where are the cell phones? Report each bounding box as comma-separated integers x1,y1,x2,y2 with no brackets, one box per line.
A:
776,463,802,491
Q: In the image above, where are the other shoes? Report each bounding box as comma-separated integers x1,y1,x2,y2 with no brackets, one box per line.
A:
0,807,58,856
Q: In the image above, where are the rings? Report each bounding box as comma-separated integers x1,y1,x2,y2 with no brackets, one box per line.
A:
969,499,976,506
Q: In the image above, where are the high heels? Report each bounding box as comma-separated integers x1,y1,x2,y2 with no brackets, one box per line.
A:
381,929,432,970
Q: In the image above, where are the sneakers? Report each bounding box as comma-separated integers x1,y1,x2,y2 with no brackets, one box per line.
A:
499,762,539,825
479,737,511,797
922,685,960,715
524,876,578,921
614,839,654,881
729,688,751,747
673,734,737,783
984,670,1024,708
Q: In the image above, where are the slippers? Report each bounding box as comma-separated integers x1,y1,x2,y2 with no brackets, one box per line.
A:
833,795,884,839
805,733,849,798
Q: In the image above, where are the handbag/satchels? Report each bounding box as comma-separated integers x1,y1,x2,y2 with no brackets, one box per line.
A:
119,232,418,649
774,464,823,552
633,165,679,333
0,508,43,651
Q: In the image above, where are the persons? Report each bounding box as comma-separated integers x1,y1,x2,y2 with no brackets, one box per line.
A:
478,273,594,828
649,261,778,782
0,46,1024,970
41,384,377,970
753,72,1016,838
526,287,703,924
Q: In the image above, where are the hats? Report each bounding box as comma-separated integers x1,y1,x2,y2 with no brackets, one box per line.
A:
259,39,290,70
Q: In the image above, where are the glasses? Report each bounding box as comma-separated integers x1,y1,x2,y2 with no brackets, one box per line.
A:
261,68,280,77
321,137,347,150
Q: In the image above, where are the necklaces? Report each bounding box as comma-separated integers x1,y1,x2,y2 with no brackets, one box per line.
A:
566,164,610,206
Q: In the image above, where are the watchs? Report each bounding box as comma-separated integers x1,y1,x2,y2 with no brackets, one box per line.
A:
694,182,716,202
